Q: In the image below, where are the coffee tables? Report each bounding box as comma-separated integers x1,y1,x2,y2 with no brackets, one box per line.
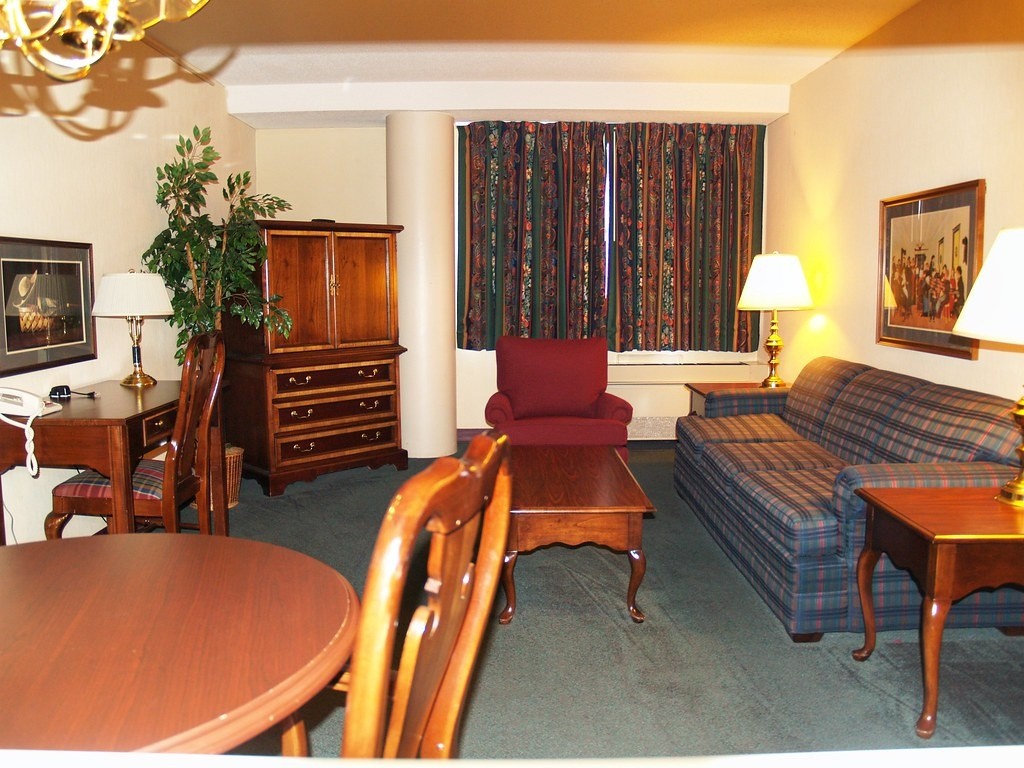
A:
496,444,658,624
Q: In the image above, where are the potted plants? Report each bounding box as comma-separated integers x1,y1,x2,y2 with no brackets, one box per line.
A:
140,125,293,510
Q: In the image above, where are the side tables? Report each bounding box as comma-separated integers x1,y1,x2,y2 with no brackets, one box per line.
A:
854,486,1024,738
685,382,792,415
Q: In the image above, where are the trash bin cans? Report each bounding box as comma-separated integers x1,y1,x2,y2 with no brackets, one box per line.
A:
190,446,245,511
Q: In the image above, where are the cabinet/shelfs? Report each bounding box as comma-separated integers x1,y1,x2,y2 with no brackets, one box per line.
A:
216,220,408,496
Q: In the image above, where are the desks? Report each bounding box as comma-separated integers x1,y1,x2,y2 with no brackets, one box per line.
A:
0,532,359,755
0,379,228,545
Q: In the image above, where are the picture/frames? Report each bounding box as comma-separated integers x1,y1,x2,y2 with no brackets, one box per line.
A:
0,235,98,379
876,179,985,361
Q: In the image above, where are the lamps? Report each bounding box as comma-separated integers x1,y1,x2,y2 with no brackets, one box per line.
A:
735,253,816,388
91,268,175,390
0,0,210,82
952,227,1024,507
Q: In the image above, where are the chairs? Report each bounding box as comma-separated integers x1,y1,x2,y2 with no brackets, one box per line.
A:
281,433,516,760
43,329,226,535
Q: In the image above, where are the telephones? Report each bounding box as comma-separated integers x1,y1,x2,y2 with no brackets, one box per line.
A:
0,387,63,417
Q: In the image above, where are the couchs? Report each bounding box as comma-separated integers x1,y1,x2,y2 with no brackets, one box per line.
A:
486,335,633,464
676,355,1024,644
